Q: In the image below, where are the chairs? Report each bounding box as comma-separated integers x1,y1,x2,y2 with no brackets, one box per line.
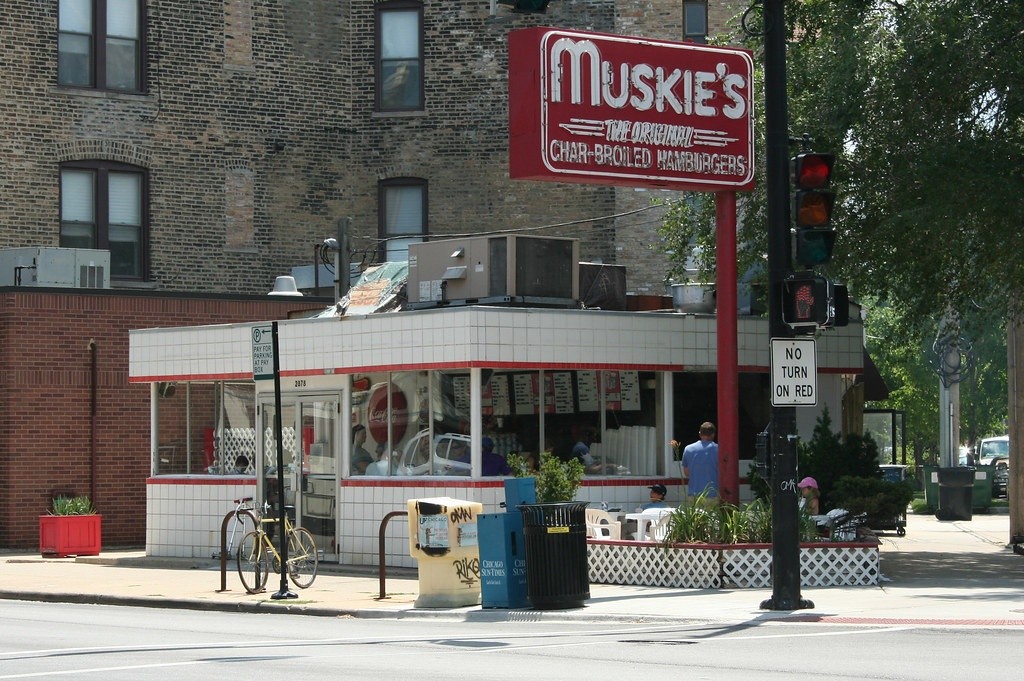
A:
587,506,868,544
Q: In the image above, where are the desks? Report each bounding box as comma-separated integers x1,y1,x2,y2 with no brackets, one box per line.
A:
625,514,664,540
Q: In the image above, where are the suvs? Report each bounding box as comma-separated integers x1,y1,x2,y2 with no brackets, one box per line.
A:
958,437,1010,498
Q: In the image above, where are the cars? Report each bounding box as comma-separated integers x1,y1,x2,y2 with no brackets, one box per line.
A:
396,427,472,475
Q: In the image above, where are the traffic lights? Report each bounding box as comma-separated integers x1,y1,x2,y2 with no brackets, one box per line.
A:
780,272,828,325
794,152,836,265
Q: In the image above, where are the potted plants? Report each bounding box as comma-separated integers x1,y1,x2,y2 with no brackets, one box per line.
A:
38,494,103,558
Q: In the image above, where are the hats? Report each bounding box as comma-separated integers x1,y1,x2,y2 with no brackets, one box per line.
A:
647,484,667,496
797,476,818,489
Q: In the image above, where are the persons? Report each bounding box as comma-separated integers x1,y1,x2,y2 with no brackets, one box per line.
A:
569,427,615,474
681,422,718,507
230,454,270,475
640,484,668,510
798,477,818,515
352,422,374,475
451,437,554,476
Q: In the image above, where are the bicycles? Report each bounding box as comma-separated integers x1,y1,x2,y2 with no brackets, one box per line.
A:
236,506,319,594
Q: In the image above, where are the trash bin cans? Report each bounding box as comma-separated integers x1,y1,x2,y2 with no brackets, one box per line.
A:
516,500,590,611
921,467,996,514
935,466,977,521
